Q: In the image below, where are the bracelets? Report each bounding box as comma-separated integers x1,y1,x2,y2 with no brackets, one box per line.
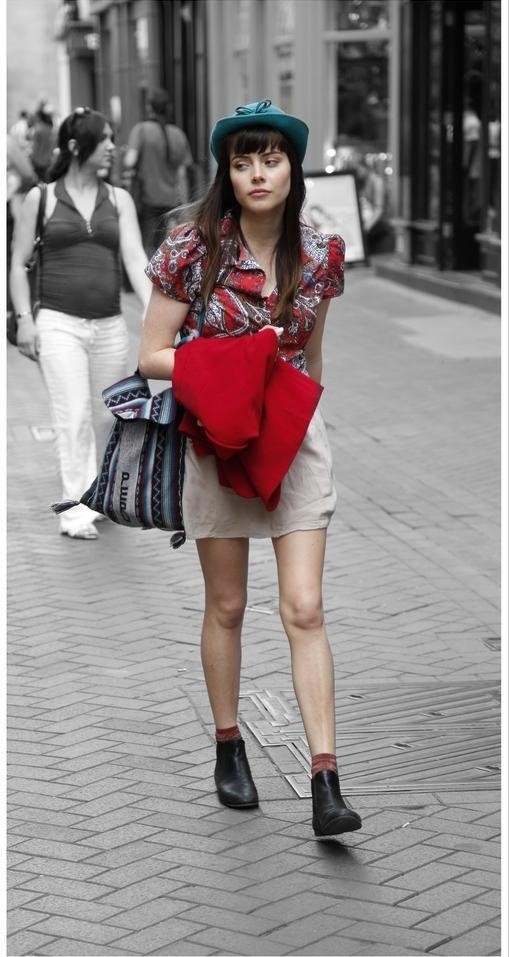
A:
15,312,30,321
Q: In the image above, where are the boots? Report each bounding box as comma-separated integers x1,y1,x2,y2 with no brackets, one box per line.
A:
310,770,363,838
212,738,260,809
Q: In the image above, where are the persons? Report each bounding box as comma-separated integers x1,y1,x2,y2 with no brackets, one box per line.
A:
124,93,194,255
8,108,154,539
135,99,363,836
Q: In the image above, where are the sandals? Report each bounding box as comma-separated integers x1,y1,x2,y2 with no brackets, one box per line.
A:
58,523,99,540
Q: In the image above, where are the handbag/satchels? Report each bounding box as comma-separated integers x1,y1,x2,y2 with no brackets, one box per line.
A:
6,181,49,347
79,372,186,532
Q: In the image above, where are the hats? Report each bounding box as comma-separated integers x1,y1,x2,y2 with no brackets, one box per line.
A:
208,97,309,166
